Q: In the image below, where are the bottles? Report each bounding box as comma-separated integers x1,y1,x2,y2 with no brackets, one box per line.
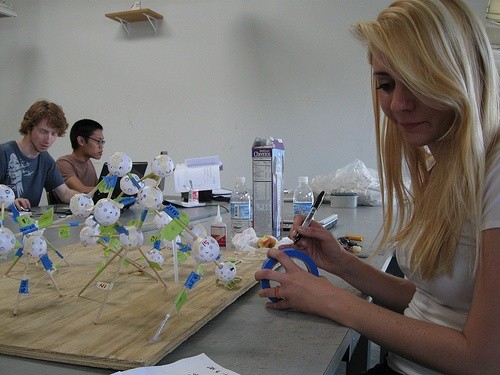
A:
229,176,253,248
292,176,315,223
210,205,227,249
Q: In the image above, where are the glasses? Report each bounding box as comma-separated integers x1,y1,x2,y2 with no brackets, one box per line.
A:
87,136,105,145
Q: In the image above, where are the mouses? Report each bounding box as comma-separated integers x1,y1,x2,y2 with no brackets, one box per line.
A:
16,205,32,212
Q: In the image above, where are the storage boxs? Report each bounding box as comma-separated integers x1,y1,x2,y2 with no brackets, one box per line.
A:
331,193,358,208
250,136,285,239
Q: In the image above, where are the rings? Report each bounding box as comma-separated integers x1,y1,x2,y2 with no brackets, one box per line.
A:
275,286,280,299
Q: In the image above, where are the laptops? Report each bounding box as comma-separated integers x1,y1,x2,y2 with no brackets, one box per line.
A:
49,161,148,210
281,214,339,231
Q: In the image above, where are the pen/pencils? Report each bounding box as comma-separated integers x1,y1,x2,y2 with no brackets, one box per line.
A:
183,179,193,191
293,191,325,245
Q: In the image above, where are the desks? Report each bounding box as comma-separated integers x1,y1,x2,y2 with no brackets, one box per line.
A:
0,196,404,375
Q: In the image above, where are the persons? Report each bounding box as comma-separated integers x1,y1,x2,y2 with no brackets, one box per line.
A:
0,101,83,211
254,0,500,375
52,119,104,203
